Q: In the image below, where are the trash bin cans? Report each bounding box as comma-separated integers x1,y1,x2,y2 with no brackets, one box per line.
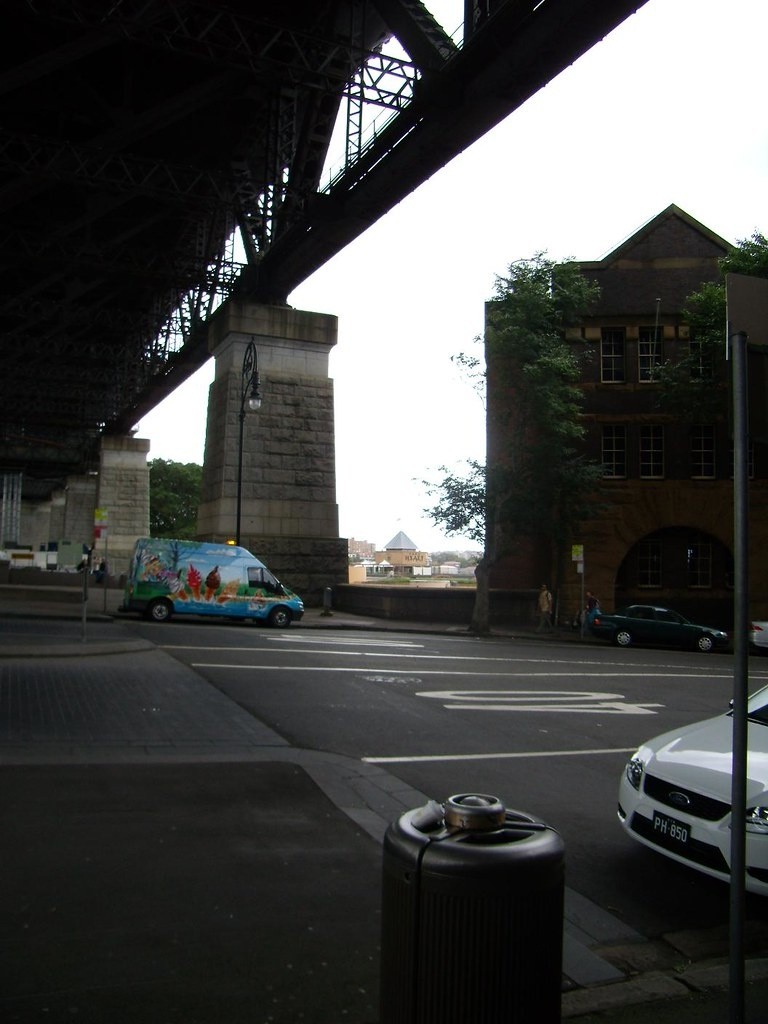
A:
378,793,566,1024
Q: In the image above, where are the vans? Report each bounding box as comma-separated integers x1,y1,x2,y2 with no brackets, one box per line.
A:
118,537,306,628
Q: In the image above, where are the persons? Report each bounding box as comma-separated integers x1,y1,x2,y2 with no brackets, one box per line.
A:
533,583,556,636
584,590,603,629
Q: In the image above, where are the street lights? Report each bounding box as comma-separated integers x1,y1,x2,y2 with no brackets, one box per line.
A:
236,337,265,545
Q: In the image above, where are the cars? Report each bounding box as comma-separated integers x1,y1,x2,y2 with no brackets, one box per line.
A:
595,604,729,654
749,620,768,648
616,682,768,902
